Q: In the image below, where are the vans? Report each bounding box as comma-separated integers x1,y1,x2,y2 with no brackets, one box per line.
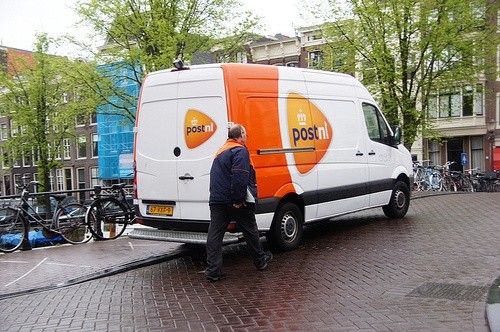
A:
128,59,413,252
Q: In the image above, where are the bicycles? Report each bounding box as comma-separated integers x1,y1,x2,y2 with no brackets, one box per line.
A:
0,180,95,252
84,180,133,242
413,158,500,194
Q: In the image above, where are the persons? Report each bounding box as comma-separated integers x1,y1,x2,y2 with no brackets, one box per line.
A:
204,123,273,281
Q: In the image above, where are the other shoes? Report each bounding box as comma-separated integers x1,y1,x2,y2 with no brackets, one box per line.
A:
257,251,273,269
206,273,230,282
